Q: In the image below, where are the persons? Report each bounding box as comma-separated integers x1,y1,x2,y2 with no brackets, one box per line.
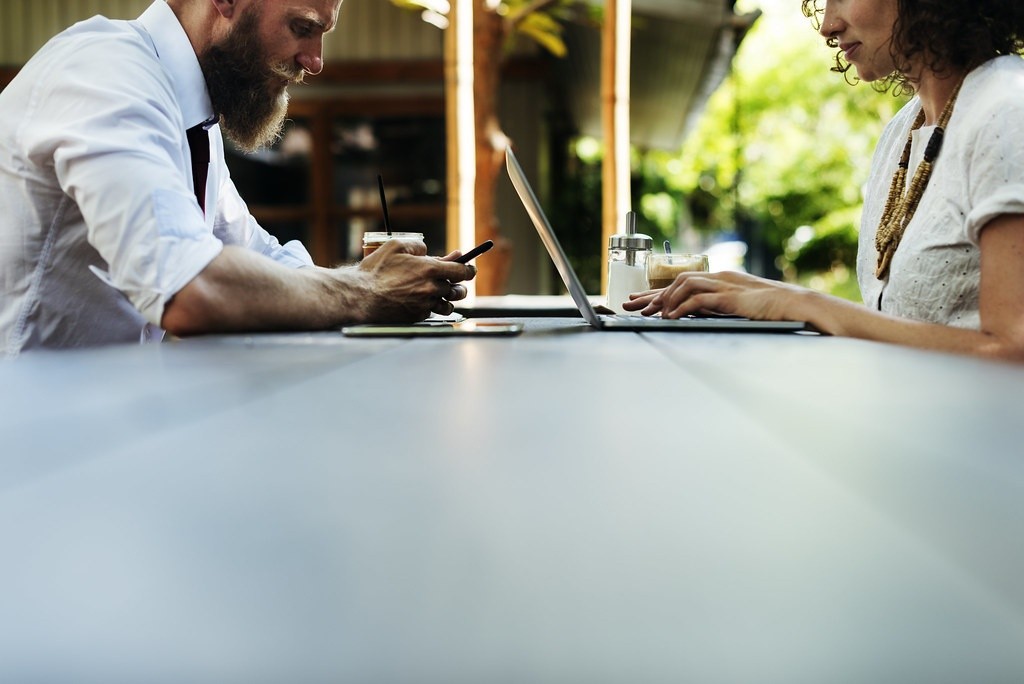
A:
1,1,477,351
623,0,1024,363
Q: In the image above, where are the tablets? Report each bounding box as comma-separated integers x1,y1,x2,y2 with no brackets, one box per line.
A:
342,322,523,336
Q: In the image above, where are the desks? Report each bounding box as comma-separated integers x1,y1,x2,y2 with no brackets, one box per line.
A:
0,309,1024,684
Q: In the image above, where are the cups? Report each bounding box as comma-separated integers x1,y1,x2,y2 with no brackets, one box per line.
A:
362,231,426,263
648,253,710,291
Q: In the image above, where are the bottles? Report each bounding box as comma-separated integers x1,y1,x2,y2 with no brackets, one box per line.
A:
603,211,653,315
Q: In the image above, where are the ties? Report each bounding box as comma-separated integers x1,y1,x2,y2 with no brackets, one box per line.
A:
186,115,220,222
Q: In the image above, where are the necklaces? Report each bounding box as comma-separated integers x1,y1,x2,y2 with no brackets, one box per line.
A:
875,73,964,280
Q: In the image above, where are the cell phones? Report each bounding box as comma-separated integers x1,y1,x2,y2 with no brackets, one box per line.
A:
452,239,494,265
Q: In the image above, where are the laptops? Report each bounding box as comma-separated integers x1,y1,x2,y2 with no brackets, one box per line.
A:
506,145,806,331
